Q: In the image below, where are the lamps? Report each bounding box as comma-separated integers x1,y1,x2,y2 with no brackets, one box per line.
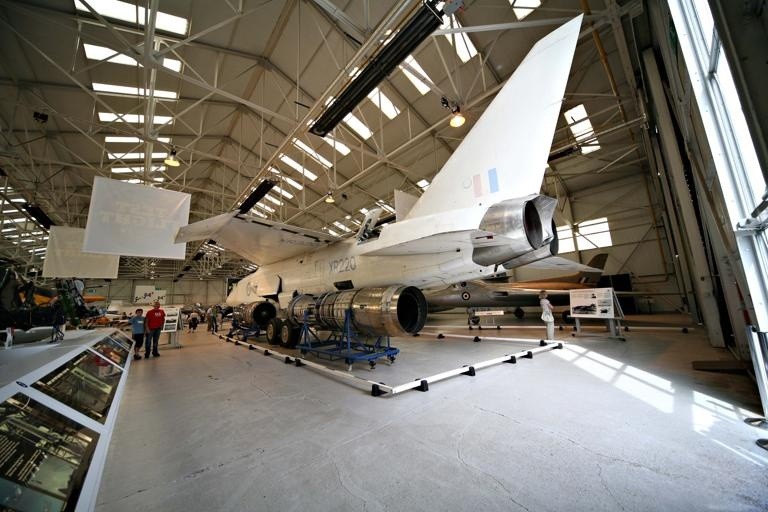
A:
324,192,335,203
449,107,465,128
150,261,156,280
163,148,180,167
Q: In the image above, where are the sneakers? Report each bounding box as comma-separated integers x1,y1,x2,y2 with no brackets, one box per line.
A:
134,352,160,359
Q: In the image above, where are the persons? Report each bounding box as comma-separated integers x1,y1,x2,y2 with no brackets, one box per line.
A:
144,301,166,358
186,303,229,335
117,309,146,360
539,290,557,340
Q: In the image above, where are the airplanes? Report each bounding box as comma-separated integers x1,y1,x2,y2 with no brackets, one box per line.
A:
180,302,214,320
420,251,609,318
172,9,607,350
20,285,107,308
